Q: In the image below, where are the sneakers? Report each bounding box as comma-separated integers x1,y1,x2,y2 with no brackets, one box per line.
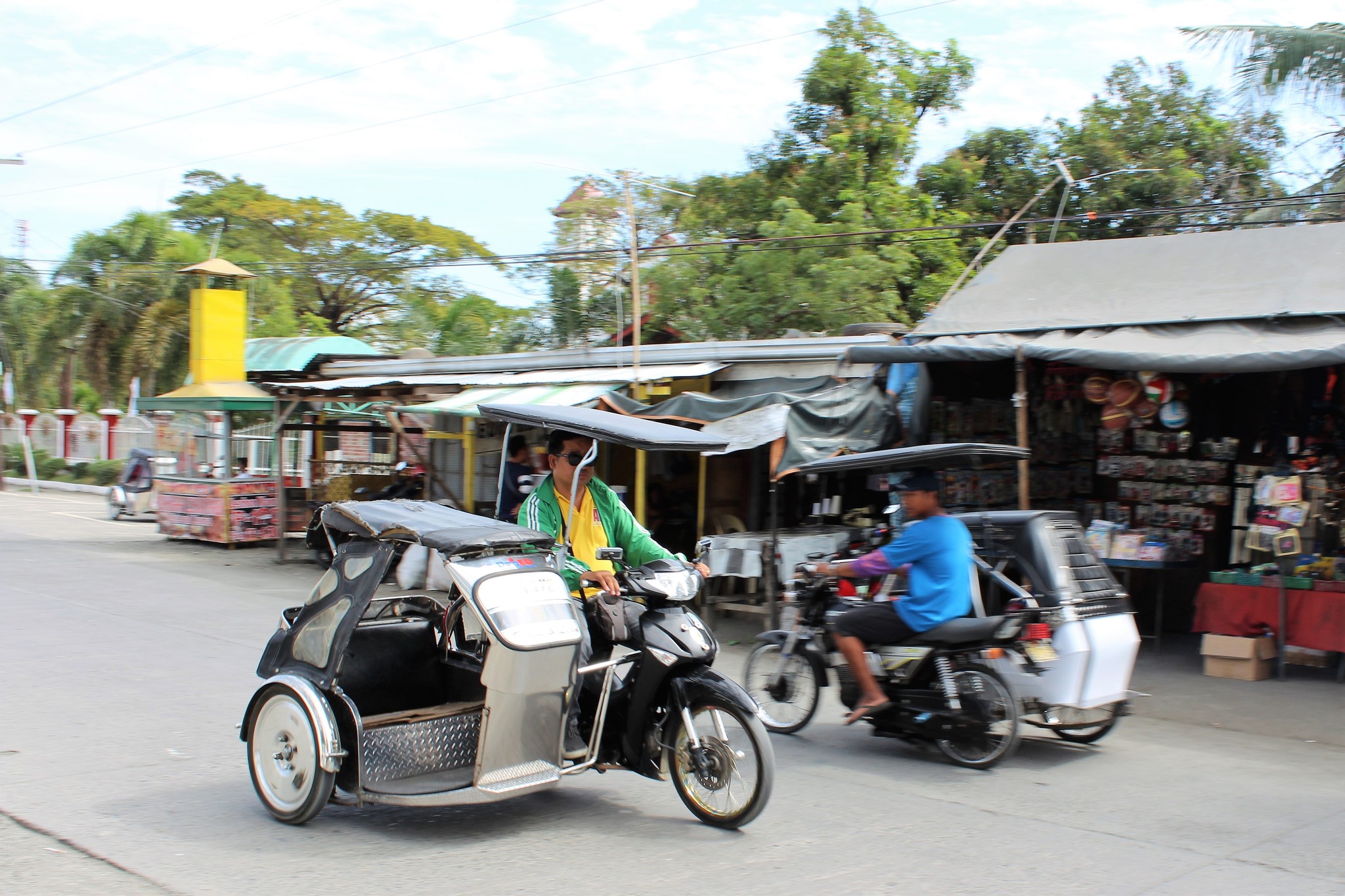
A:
562,718,588,759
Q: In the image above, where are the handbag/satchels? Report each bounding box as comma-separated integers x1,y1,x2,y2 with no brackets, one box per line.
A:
583,591,632,648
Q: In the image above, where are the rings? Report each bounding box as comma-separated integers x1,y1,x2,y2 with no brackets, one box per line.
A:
707,570,710,572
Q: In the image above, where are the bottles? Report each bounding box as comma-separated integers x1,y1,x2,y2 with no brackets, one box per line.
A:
197,463,209,473
208,463,213,471
1312,553,1321,563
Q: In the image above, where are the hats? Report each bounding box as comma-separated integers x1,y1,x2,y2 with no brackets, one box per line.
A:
884,465,939,493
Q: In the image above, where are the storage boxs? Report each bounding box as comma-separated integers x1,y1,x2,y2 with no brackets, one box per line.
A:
1211,563,1345,591
1203,631,1279,682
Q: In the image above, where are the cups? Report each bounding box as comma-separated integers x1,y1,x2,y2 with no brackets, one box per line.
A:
352,466,357,473
812,503,821,515
830,496,842,514
821,499,831,514
380,466,385,473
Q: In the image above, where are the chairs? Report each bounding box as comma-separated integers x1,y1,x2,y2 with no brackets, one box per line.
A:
713,515,757,605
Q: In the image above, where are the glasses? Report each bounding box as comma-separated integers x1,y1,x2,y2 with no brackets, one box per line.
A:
548,450,599,467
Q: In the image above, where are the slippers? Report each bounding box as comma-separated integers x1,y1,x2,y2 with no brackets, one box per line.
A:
838,694,890,726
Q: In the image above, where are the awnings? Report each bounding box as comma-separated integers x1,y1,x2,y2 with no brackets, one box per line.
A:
382,383,630,512
846,312,1345,373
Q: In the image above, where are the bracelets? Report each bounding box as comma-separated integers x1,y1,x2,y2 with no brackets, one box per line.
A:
826,564,839,576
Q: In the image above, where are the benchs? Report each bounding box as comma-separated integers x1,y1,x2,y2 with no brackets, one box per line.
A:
321,610,465,720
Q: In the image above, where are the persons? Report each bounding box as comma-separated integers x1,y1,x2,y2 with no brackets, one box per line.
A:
234,457,251,478
817,473,973,725
516,428,711,764
498,434,535,525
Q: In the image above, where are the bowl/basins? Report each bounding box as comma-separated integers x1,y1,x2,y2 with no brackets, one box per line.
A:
323,479,330,485
324,449,343,474
314,480,322,485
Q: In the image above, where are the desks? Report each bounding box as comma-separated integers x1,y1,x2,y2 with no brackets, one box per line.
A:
699,526,854,634
1194,580,1345,665
1099,557,1186,641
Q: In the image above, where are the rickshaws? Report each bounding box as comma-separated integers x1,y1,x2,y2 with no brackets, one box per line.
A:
238,404,776,830
108,450,180,521
740,444,1140,771
